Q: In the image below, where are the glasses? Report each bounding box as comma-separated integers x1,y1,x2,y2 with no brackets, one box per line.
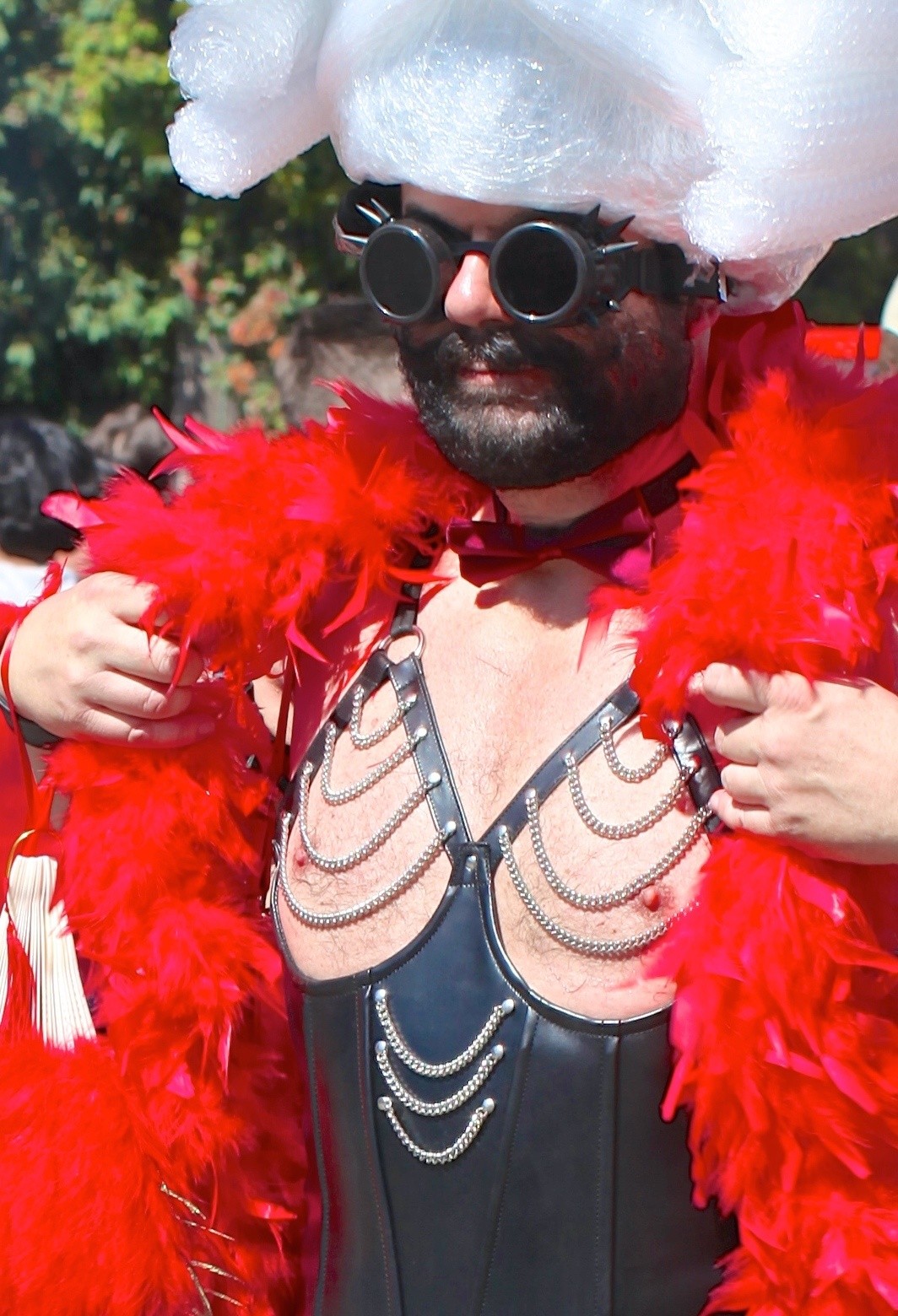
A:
334,192,732,334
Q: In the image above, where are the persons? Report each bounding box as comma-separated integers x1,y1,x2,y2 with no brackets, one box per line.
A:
0,3,898,1316
0,401,168,606
